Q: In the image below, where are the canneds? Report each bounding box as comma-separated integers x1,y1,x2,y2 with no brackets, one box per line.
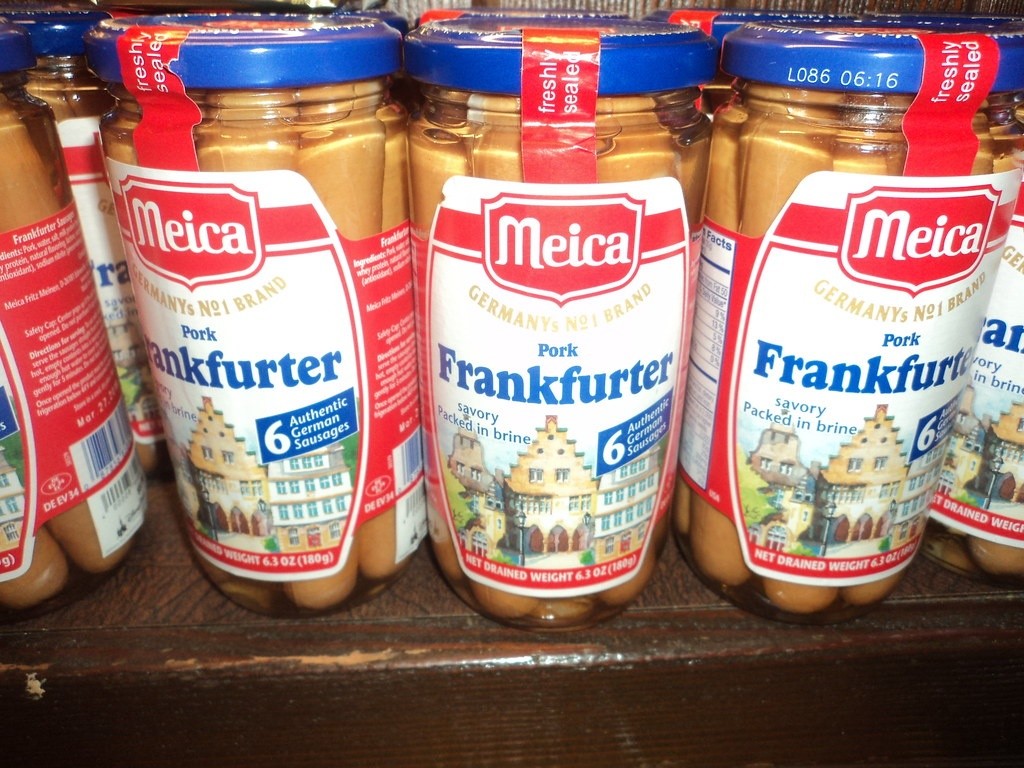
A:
0,0,1024,632
